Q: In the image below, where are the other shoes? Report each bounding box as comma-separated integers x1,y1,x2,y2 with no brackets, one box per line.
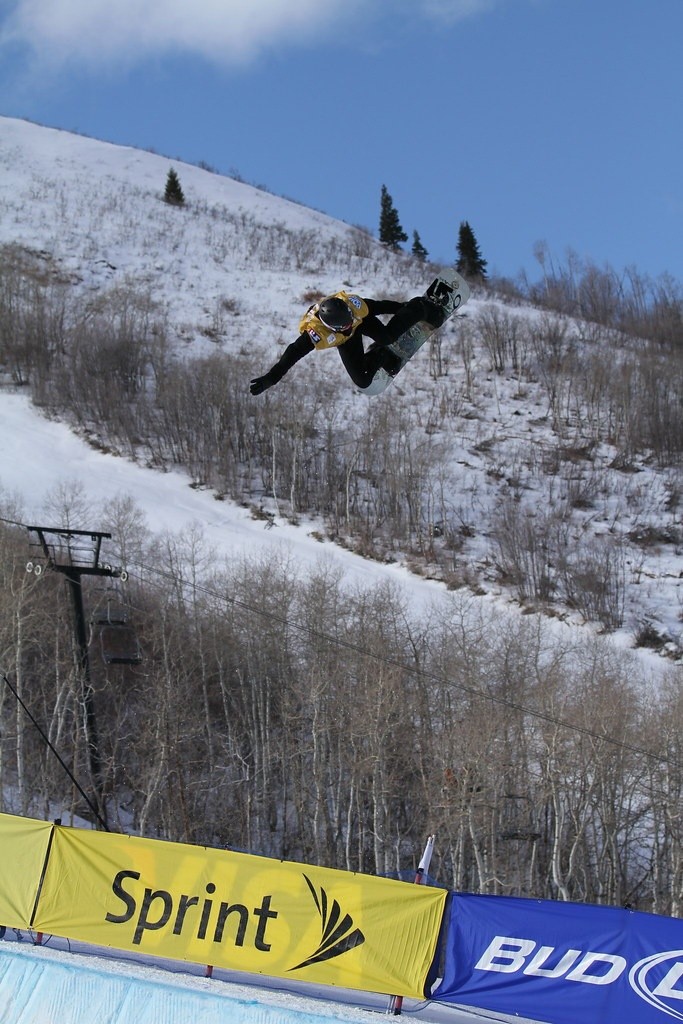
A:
413,297,444,321
378,346,400,375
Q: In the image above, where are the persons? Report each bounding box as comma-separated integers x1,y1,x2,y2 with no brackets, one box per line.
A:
250,292,444,395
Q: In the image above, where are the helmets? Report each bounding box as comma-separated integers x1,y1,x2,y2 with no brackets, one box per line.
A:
319,297,353,330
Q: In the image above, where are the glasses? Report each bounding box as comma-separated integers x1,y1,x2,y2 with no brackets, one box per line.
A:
339,319,353,331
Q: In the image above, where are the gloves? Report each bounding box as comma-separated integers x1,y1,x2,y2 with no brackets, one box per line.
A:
250,375,273,395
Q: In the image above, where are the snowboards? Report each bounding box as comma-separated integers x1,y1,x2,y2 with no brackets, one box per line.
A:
355,267,470,396
